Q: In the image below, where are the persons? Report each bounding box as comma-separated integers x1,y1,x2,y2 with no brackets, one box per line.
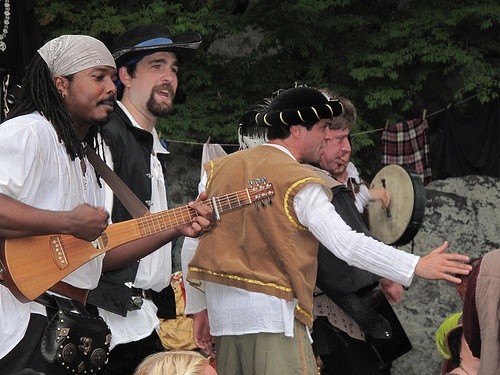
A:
181,84,473,375
96,23,202,375
0,34,217,375
431,248,500,375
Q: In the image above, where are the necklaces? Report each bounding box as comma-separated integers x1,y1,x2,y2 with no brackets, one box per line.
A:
460,366,470,375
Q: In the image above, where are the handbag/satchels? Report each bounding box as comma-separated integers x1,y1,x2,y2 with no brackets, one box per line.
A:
41,308,112,375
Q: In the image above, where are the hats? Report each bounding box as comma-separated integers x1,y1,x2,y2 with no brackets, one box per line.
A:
253,87,344,127
111,24,202,65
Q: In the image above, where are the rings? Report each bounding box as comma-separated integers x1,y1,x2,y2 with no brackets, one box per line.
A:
203,226,211,232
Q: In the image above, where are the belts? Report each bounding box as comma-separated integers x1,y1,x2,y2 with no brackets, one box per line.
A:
48,280,90,307
131,286,156,300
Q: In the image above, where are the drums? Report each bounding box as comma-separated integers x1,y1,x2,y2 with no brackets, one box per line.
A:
368,165,425,247
